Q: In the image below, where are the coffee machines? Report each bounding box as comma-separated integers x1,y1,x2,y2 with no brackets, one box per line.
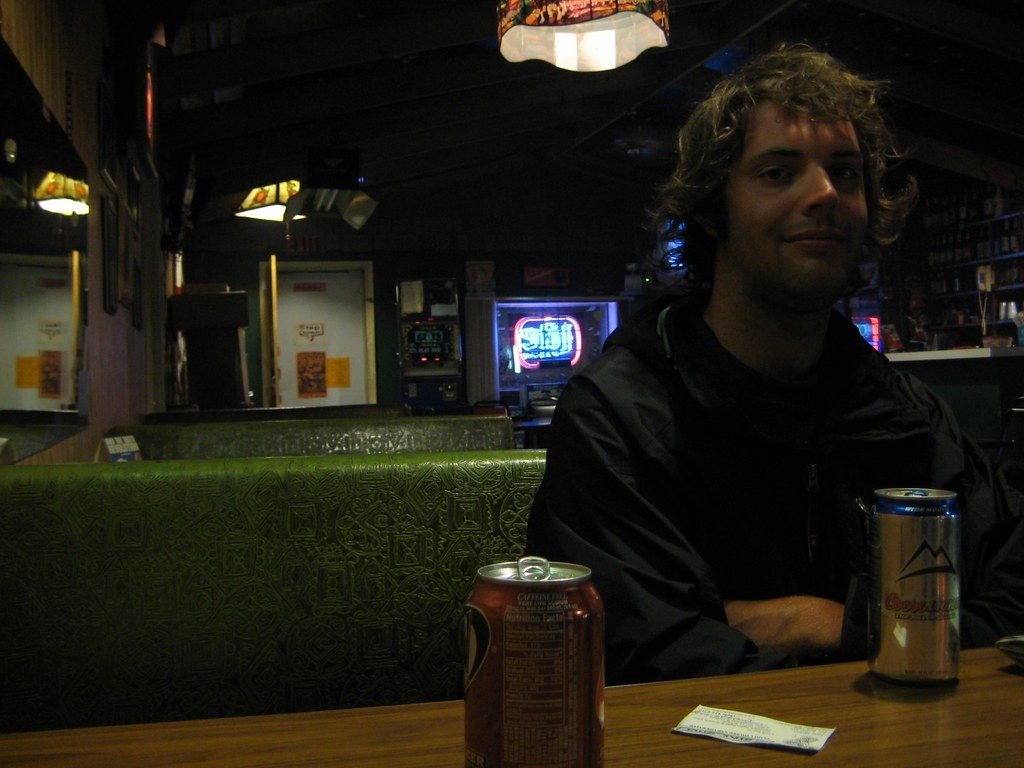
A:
394,271,466,410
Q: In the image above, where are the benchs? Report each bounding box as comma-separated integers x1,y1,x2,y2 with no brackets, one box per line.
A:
1,402,553,726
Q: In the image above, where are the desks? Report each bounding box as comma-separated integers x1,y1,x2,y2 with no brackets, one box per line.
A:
0,643,1024,768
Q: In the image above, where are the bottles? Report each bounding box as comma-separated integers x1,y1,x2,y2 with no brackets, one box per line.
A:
901,171,1024,354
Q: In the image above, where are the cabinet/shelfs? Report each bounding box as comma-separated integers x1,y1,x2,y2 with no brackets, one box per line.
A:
910,188,1024,350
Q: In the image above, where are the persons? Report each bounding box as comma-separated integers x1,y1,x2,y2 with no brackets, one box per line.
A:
527,42,1024,685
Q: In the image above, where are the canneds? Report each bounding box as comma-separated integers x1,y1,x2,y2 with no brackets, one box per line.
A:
457,557,607,768
861,488,963,684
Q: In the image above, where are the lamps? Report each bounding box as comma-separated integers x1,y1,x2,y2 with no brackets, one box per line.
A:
234,179,308,222
495,0,672,72
36,172,91,216
